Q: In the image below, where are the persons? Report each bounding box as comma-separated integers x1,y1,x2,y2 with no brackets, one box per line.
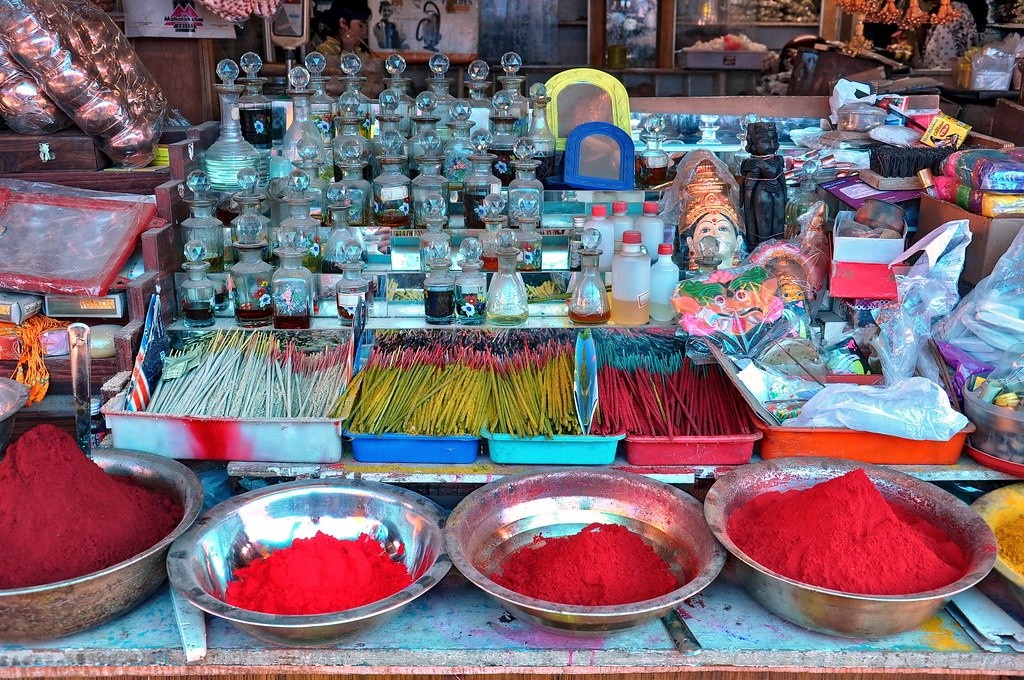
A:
930,124,958,148
738,122,788,242
373,1,406,49
314,0,389,77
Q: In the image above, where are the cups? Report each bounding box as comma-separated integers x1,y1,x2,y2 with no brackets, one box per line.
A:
608,45,627,70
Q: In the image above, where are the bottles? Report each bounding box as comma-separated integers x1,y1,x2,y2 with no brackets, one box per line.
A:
179,168,320,272
640,114,668,187
608,201,635,254
334,241,368,327
650,243,680,321
89,398,109,449
583,204,613,271
230,214,276,329
204,51,370,226
273,225,314,329
565,218,585,305
419,192,543,325
321,182,362,273
569,228,611,325
371,52,555,229
611,230,651,326
635,202,664,260
785,161,821,226
177,241,216,328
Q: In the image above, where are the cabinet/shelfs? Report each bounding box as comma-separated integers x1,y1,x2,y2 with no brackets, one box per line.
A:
457,0,857,97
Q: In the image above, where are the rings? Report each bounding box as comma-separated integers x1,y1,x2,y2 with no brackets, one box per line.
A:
347,34,349,38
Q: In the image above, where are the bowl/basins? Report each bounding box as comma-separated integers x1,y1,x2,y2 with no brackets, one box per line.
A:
962,370,1024,465
836,103,886,131
0,377,30,457
445,468,725,637
0,446,203,638
165,477,455,648
704,456,999,638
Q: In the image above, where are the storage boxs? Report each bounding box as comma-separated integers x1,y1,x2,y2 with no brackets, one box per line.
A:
919,113,973,149
0,329,70,360
677,50,768,69
101,326,1024,465
1,125,109,174
368,0,480,62
969,71,1013,91
1,289,126,326
815,195,1024,340
